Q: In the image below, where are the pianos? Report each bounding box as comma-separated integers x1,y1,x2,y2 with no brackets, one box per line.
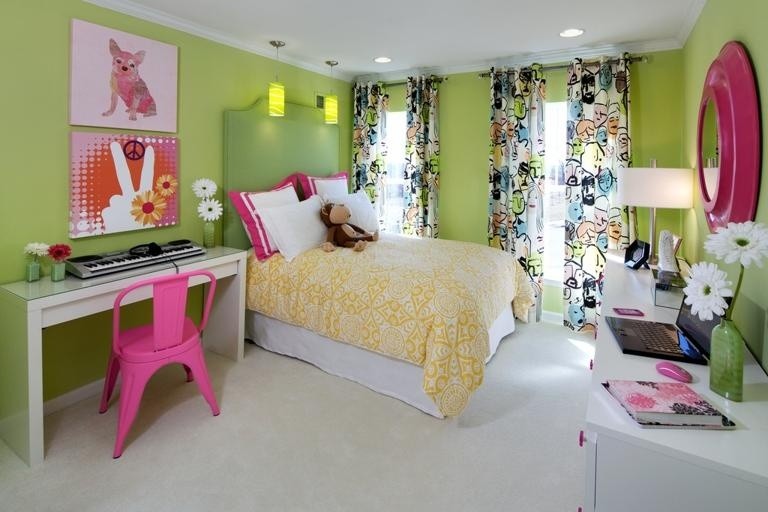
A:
62,239,208,280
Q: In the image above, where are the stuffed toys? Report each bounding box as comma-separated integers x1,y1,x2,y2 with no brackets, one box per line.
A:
320,203,379,252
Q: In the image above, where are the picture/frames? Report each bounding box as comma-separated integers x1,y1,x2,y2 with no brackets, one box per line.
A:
69,18,179,133
66,131,180,240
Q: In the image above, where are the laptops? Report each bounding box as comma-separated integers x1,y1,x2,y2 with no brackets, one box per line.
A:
605,246,746,365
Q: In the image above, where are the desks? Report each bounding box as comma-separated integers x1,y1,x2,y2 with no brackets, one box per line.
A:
586,250,768,511
0,244,247,469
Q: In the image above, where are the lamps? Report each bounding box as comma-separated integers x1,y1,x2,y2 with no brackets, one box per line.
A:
325,61,338,123
373,57,392,63
560,28,584,38
616,158,695,263
268,40,286,116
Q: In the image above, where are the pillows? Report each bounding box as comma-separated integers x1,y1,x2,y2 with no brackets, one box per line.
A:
254,194,327,263
227,174,297,262
320,189,380,231
297,172,348,199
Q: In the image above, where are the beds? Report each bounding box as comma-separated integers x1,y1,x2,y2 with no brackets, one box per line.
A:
242,231,536,422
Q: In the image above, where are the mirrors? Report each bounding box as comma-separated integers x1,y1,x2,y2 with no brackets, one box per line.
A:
694,40,761,235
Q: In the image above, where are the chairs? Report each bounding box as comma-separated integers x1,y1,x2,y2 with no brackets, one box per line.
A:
98,270,220,459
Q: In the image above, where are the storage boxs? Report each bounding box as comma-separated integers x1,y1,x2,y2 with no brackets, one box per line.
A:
649,268,685,309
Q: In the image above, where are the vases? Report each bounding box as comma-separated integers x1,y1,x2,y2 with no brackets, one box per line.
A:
709,319,743,402
51,262,65,281
26,263,40,282
203,221,215,249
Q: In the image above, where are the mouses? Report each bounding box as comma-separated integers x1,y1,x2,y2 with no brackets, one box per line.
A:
656,362,692,383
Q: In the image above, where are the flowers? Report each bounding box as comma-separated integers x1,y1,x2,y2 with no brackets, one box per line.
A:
48,244,72,263
155,174,177,197
192,177,224,221
130,189,167,226
683,220,768,321
24,243,50,263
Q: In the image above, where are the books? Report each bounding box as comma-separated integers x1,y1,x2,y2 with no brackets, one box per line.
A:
601,380,736,430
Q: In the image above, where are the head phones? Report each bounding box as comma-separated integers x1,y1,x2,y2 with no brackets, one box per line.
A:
129,242,161,257
